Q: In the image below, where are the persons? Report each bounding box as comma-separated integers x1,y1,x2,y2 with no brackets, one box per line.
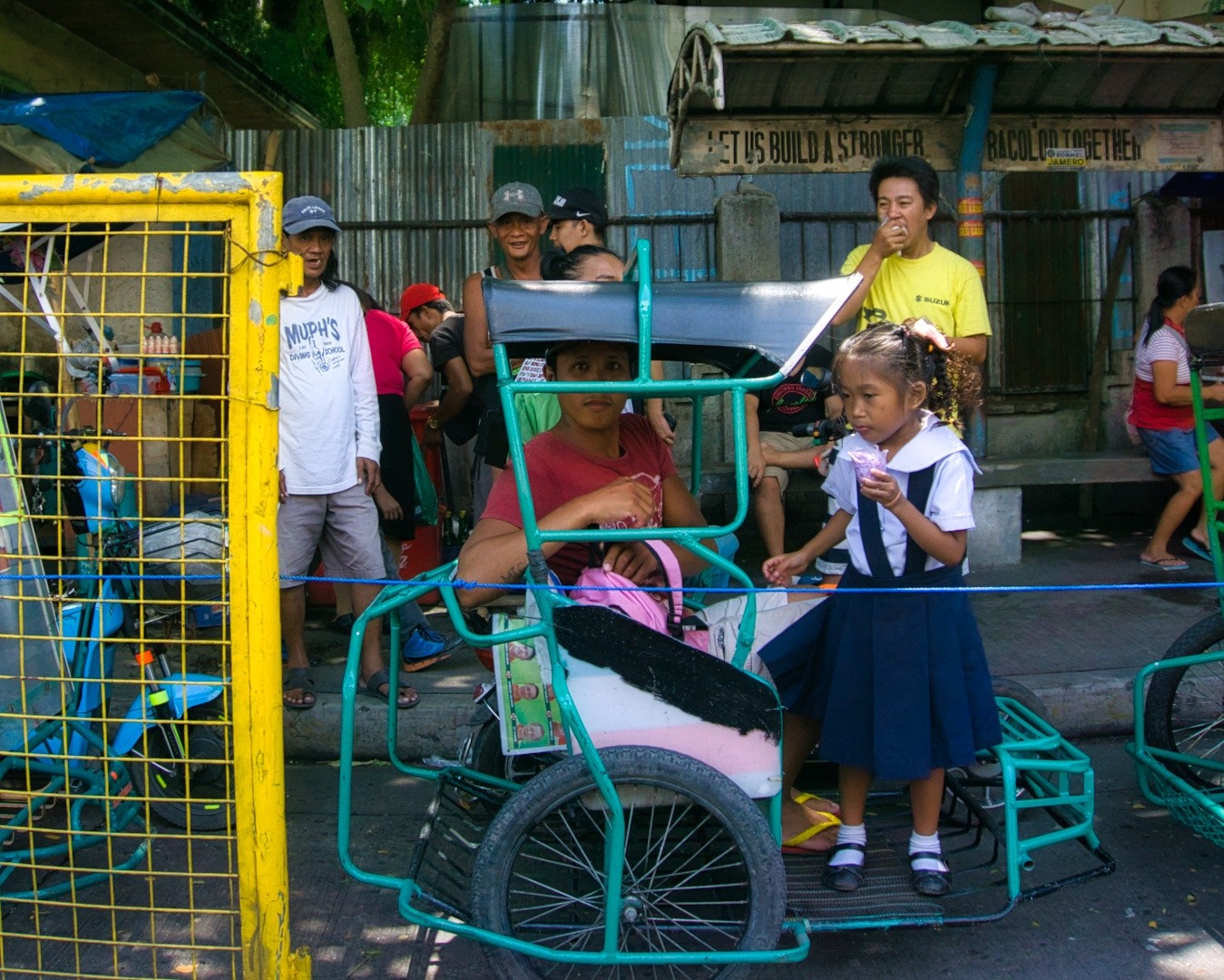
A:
455,340,841,857
515,243,629,448
276,192,423,714
463,180,547,483
1129,265,1224,566
830,157,992,440
546,185,676,448
763,318,1000,896
319,284,480,674
745,342,844,588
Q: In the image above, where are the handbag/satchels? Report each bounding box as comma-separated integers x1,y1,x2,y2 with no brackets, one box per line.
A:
440,389,478,447
411,425,439,527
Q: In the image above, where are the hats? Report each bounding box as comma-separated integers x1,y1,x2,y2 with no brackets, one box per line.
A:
549,188,609,226
284,195,343,235
490,181,544,223
400,284,446,321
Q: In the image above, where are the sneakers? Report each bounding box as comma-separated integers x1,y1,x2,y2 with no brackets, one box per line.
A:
402,626,464,672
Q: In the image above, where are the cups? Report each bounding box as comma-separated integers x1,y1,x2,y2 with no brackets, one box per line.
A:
118,344,140,353
849,451,887,491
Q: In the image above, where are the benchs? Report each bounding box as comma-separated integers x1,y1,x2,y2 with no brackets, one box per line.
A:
520,561,782,810
675,458,1166,569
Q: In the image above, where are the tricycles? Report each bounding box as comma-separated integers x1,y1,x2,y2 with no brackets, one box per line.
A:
1,216,232,904
1124,301,1222,852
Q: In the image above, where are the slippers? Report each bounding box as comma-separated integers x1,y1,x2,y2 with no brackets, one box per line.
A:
282,666,317,709
1140,554,1189,571
794,791,841,817
357,667,420,709
1182,536,1214,562
781,811,843,854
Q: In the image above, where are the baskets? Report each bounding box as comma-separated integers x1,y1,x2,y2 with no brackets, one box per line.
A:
105,512,229,616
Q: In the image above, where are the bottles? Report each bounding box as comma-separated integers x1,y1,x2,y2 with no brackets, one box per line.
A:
143,322,178,354
441,509,470,548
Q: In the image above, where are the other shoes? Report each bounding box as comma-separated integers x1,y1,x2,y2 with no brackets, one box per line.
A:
906,849,953,895
823,842,867,891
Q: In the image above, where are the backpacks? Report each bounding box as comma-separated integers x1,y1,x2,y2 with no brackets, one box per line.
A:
572,539,711,652
